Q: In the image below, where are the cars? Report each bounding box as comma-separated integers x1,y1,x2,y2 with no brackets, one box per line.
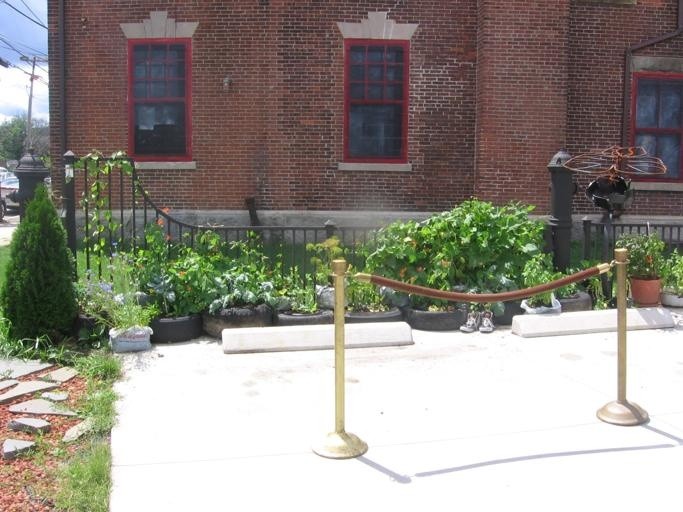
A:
0,177,21,217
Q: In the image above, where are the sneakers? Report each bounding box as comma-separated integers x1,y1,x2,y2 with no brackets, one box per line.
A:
479,310,495,332
459,311,480,333
